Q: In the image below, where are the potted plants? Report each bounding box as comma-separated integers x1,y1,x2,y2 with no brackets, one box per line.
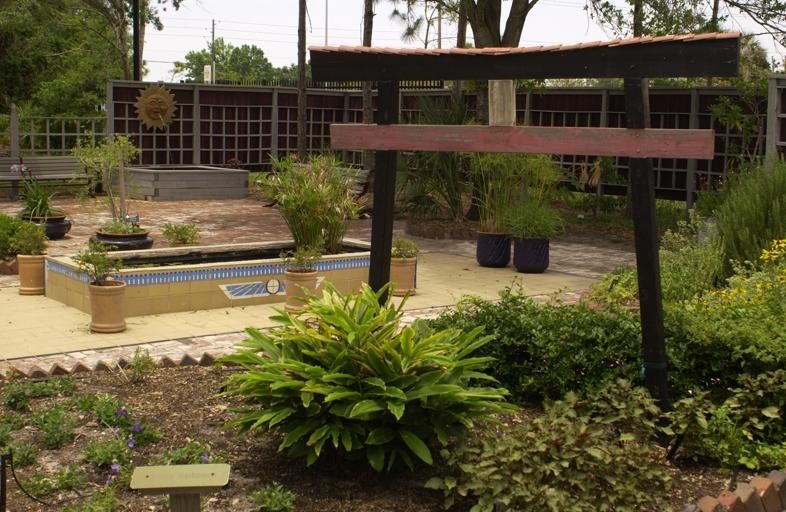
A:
465,147,569,274
0,210,23,276
8,220,49,296
16,174,72,240
389,233,420,296
252,145,368,318
70,235,128,334
70,132,154,252
